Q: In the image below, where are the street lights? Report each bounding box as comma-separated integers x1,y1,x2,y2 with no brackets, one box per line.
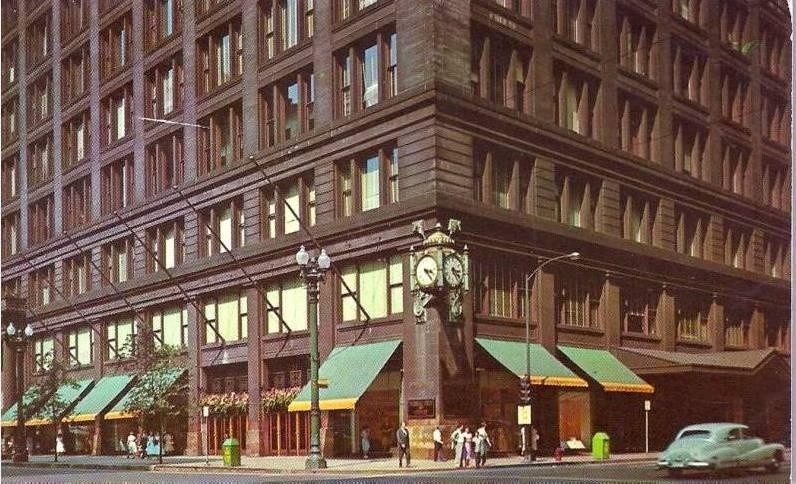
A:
523,252,581,461
2,321,33,462
295,244,331,469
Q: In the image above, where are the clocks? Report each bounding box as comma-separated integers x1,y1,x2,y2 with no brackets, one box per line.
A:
414,254,464,288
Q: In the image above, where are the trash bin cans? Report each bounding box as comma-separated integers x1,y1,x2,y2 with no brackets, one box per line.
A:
222,438,240,466
591,431,610,460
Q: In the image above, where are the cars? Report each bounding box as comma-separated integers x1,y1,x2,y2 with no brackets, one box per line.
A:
654,422,785,478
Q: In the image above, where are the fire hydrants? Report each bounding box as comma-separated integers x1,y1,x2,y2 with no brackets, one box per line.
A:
554,447,564,461
